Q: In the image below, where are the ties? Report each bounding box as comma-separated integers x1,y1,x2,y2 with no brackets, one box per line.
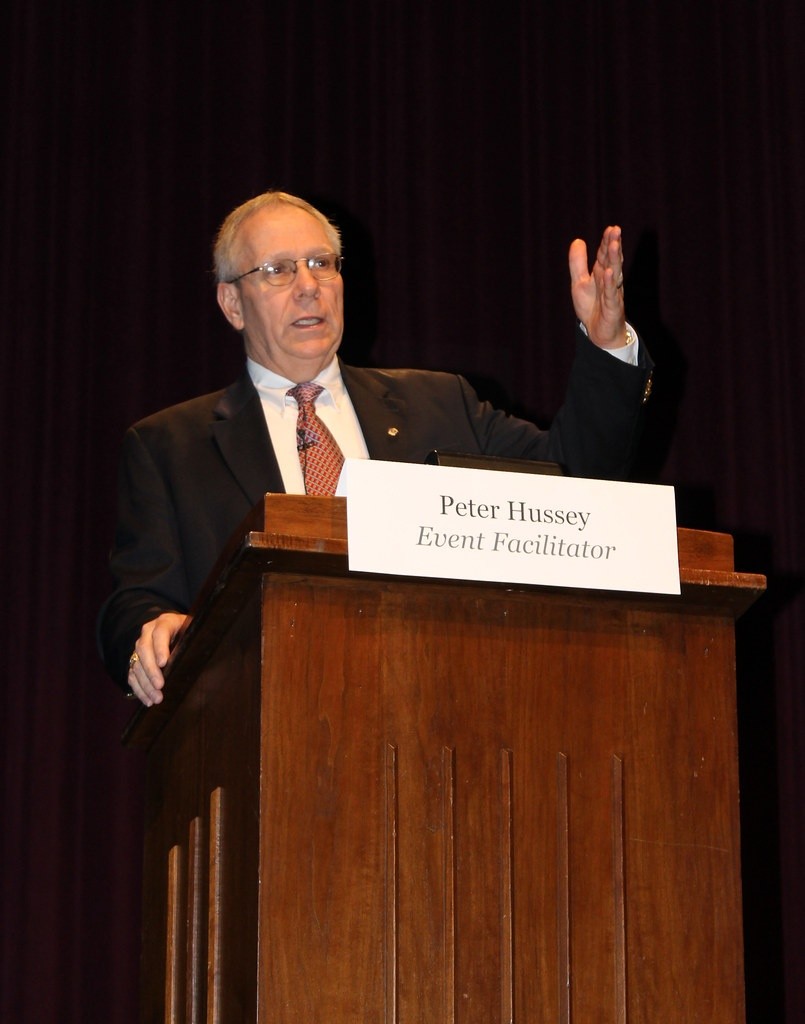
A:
285,381,346,498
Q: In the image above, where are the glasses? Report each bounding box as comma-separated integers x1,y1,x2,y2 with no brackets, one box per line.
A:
227,253,344,286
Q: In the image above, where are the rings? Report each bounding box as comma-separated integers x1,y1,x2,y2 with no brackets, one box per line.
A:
616,280,623,288
129,654,138,669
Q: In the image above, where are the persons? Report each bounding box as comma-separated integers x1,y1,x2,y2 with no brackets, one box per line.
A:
95,190,652,707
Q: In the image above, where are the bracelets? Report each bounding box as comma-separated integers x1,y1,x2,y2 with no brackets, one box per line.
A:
625,329,632,345
